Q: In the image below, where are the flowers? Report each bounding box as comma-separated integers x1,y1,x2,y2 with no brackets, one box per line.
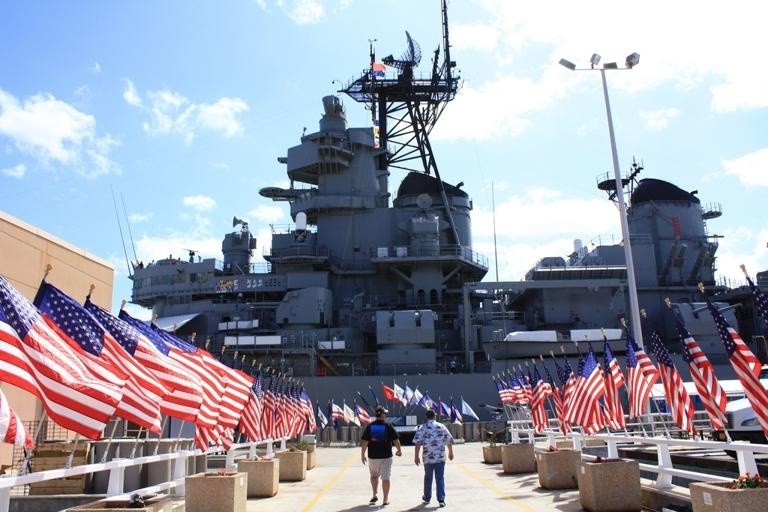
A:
723,468,766,490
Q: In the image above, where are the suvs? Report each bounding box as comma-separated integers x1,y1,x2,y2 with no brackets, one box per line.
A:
709,396,768,467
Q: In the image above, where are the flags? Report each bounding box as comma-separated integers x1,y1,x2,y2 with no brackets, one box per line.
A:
1,388,36,449
117,308,224,431
462,400,479,420
358,380,463,426
0,276,126,443
34,280,168,436
83,300,205,424
372,62,387,78
494,262,768,447
315,398,369,431
153,324,317,452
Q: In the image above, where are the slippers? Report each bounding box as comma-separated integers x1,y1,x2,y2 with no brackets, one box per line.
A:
370,496,378,502
383,501,390,505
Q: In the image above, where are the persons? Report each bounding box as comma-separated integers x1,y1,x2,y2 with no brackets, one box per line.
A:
361,405,402,507
412,409,455,507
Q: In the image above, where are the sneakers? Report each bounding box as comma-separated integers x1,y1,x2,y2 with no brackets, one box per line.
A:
422,495,430,503
439,500,446,507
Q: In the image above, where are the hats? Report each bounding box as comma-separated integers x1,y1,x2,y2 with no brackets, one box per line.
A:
425,410,435,418
376,405,389,416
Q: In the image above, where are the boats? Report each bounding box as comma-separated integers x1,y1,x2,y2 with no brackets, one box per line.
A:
111,0,768,425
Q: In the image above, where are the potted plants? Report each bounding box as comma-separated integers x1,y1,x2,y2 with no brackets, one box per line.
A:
293,435,316,469
479,430,504,464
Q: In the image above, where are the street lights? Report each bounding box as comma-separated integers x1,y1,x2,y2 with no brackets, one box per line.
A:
558,49,655,432
